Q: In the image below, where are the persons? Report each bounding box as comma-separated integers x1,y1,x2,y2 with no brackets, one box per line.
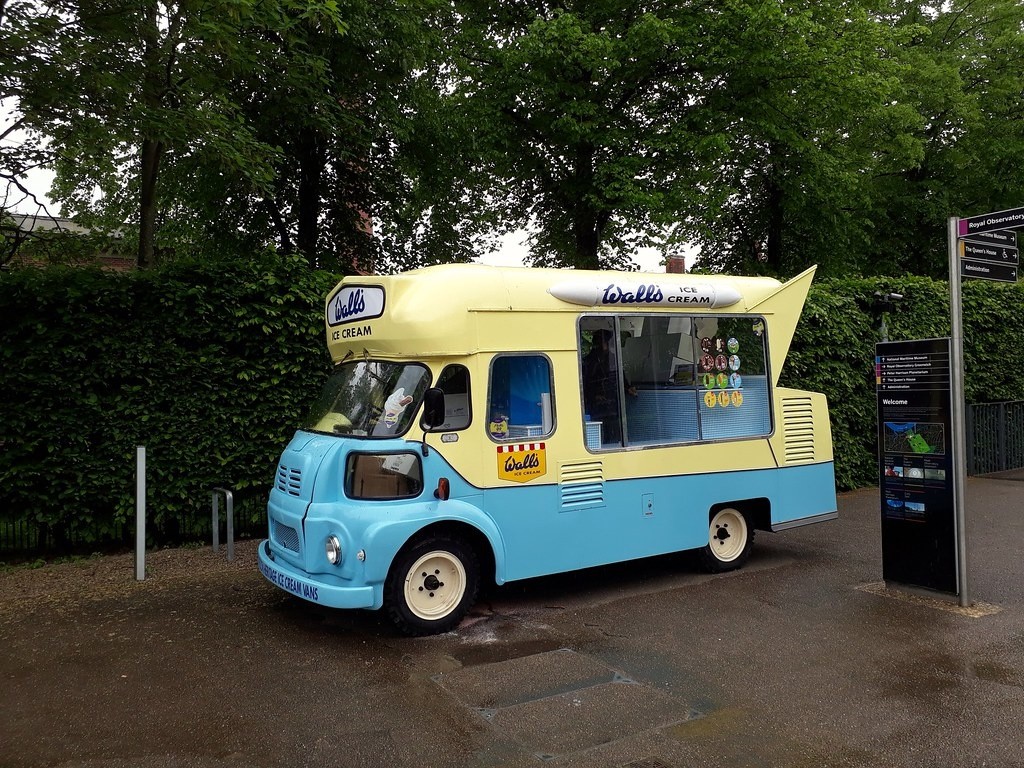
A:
368,388,409,433
582,329,638,443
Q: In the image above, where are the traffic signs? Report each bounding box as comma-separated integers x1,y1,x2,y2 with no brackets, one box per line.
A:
957,206,1024,238
961,259,1018,282
958,241,1019,265
959,229,1017,248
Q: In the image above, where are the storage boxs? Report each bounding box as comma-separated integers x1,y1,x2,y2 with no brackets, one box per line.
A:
626,317,702,384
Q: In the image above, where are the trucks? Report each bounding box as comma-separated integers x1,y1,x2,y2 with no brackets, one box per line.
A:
259,262,840,636
